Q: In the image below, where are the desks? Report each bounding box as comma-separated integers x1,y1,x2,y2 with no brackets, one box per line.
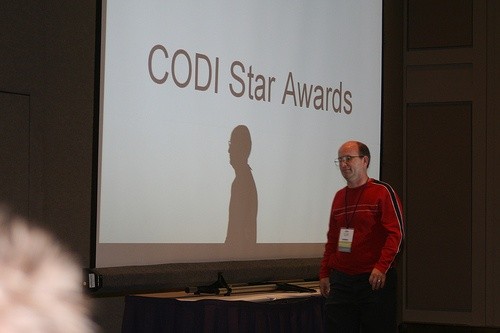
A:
119,279,330,333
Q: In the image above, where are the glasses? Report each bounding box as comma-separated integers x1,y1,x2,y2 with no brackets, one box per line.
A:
334,155,366,166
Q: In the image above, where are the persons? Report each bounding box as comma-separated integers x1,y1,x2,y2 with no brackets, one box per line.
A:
320,141,404,333
